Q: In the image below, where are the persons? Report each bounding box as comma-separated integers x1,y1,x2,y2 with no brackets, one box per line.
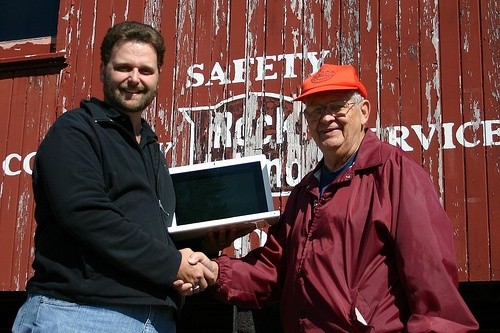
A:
11,21,216,333
173,63,480,333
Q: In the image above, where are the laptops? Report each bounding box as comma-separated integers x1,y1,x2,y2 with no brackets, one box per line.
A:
167,154,280,242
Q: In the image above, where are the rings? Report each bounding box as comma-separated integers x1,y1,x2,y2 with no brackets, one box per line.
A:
194,285,200,290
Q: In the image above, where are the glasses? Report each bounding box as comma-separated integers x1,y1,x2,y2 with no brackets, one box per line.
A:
301,100,356,121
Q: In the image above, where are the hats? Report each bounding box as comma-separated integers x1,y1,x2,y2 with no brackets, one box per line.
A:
292,63,368,100
